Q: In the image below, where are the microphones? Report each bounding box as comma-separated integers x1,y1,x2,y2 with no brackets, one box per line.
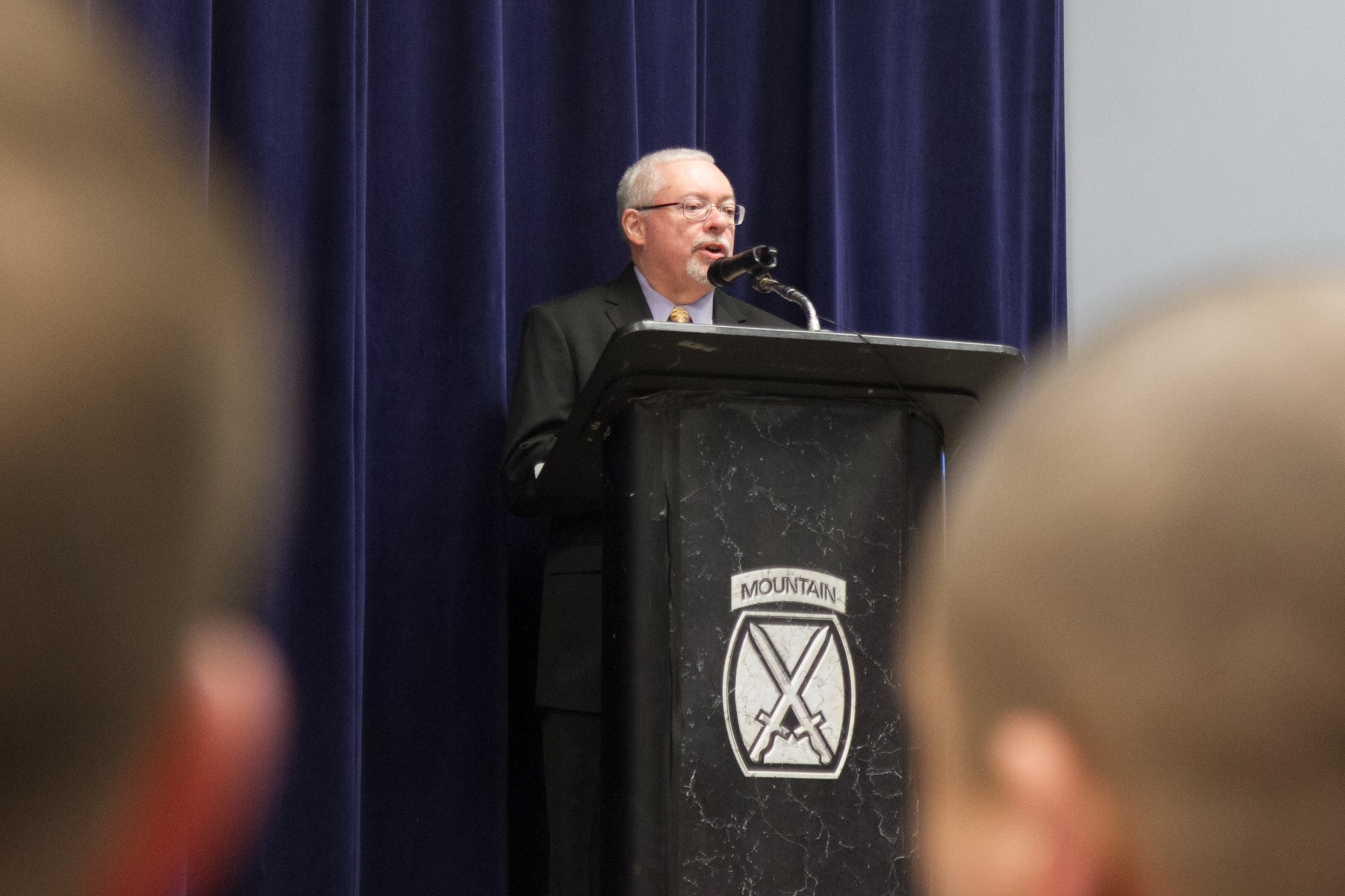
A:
707,245,779,287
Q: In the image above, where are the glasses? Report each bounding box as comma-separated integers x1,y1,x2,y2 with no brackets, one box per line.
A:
635,198,746,226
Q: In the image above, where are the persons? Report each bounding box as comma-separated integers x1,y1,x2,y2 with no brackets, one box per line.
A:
893,261,1345,896
503,148,801,480
0,1,292,896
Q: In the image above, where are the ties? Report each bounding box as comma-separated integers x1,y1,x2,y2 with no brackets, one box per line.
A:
670,308,692,325
